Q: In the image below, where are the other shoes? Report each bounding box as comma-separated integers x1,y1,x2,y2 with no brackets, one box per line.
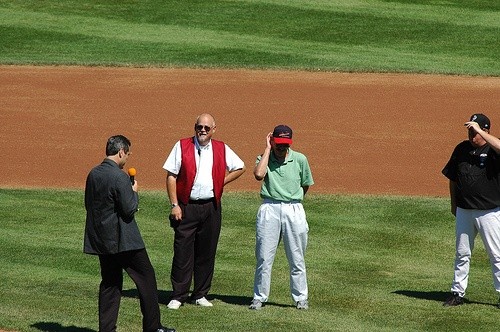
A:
167,300,181,310
191,296,213,306
156,325,176,332
442,291,463,307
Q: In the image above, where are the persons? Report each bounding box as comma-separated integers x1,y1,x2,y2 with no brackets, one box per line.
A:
83,135,175,332
441,113,500,307
162,114,246,309
249,125,314,310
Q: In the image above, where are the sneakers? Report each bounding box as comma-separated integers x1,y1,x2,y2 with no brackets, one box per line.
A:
248,299,265,309
297,300,309,310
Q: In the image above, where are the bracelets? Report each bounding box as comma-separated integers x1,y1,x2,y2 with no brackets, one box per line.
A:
171,203,178,209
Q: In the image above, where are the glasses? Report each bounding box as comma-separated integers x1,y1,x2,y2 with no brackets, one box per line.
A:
197,125,214,132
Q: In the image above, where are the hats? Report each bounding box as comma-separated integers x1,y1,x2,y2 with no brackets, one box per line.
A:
272,125,293,144
463,113,491,131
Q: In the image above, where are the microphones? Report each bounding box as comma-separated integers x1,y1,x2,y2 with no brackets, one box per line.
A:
128,167,137,186
